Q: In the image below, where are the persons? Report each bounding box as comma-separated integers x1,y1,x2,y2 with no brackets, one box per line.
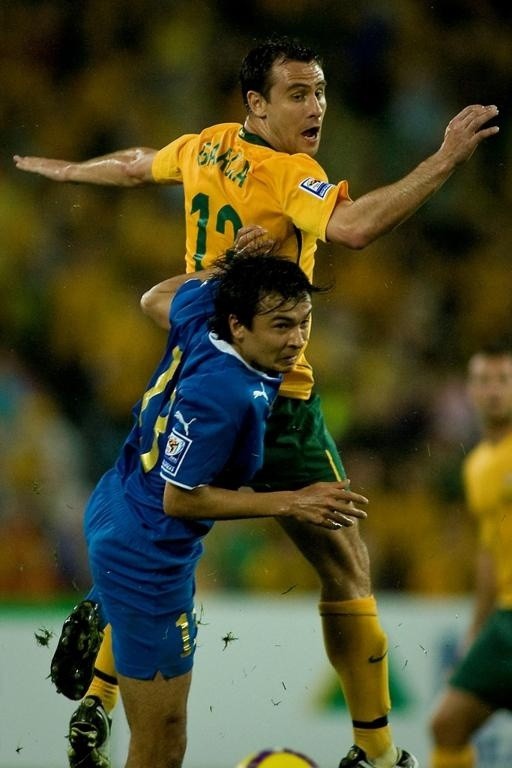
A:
67,224,370,767
429,342,512,767
13,37,499,768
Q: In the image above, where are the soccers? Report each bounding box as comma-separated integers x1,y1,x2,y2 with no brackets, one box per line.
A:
234,747,319,768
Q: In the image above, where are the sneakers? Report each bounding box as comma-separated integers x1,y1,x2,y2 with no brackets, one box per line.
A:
340,746,418,768
51,601,104,700
66,696,112,768
